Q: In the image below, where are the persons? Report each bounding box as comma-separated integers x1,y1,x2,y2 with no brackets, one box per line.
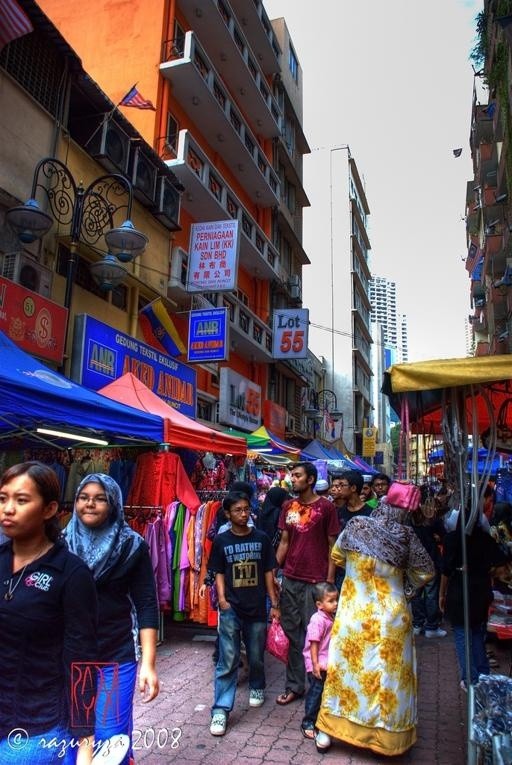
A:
1,460,511,765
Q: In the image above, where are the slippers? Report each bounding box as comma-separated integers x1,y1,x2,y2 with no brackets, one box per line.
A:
300,723,317,740
276,689,298,705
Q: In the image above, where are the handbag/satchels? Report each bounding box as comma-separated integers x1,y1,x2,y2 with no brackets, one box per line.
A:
386,479,438,528
266,620,291,666
482,423,512,453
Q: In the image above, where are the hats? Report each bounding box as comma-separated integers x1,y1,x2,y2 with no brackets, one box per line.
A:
314,478,330,492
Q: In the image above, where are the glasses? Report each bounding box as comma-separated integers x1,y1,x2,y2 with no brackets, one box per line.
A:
75,494,111,507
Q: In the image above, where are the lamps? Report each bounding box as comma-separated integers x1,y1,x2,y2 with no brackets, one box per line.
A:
30,418,112,448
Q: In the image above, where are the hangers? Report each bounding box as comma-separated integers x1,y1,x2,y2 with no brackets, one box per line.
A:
195,487,228,503
121,502,162,525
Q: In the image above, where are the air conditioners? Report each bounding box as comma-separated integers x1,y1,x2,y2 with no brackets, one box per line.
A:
290,286,303,305
287,414,296,433
288,273,301,290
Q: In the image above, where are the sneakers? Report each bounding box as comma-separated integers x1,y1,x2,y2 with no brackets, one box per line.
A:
210,710,228,737
413,626,422,636
424,627,448,640
248,684,265,707
314,730,332,753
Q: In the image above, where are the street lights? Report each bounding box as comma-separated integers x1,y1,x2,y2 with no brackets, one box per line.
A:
4,156,149,378
303,389,343,441
